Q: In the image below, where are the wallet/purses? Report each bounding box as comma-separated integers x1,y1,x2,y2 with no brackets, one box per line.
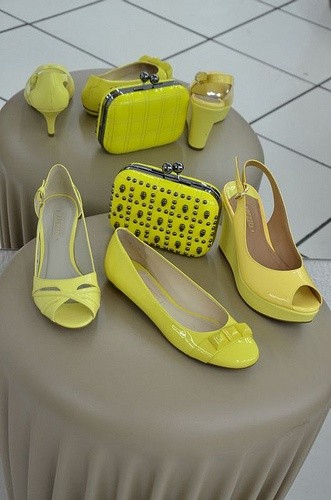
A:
96,70,190,155
109,160,223,258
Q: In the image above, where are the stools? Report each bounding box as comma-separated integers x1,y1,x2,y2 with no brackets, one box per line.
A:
0,212,331,500
1,64,263,247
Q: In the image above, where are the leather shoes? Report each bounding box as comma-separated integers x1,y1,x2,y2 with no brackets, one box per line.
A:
104,226,259,370
81,55,172,118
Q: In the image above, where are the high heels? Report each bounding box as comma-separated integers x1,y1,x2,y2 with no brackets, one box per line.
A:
23,64,75,137
218,155,323,322
185,72,233,150
34,164,101,328
